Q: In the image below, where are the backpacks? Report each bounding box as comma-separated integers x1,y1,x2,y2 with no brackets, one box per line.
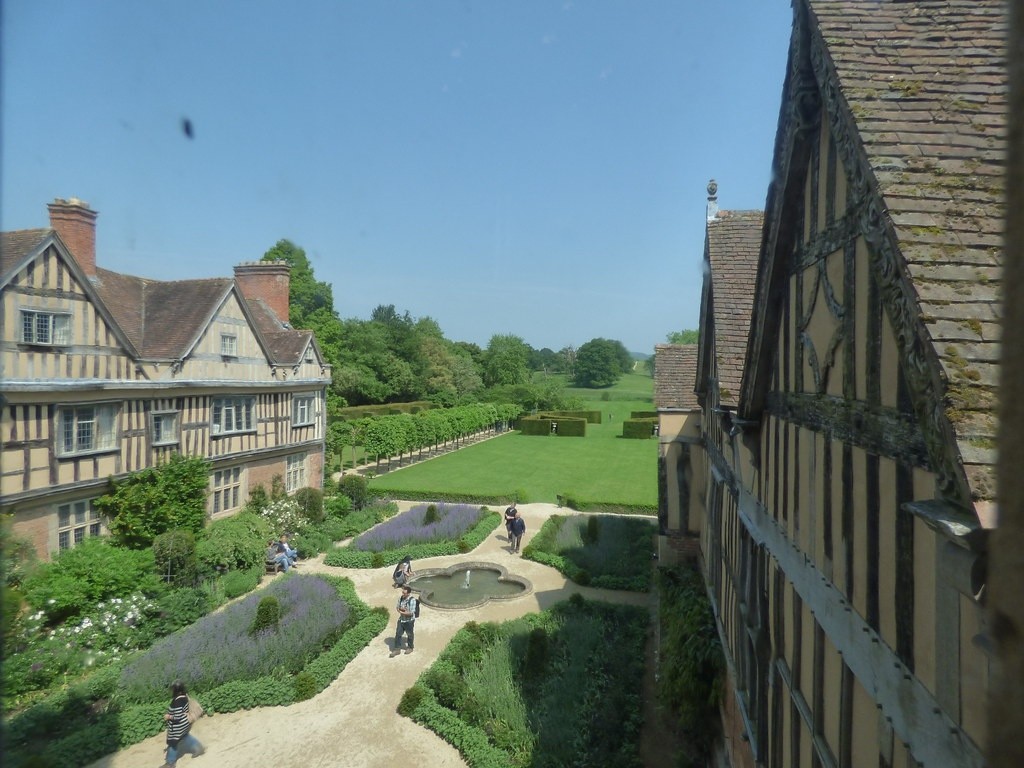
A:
400,596,420,618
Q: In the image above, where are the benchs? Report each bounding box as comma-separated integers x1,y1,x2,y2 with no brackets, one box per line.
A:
262,545,297,575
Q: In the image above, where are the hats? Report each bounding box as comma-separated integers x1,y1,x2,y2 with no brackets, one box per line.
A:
404,555,413,560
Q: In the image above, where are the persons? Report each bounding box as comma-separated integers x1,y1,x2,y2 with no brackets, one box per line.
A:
390,587,416,658
509,512,525,554
505,503,517,542
393,556,411,588
158,679,205,768
280,535,297,559
267,541,291,572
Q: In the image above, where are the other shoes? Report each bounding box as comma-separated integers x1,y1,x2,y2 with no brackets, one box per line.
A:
192,745,205,757
392,582,395,585
159,762,176,768
292,565,297,567
510,549,514,553
389,649,400,657
292,562,298,566
516,549,519,553
395,584,398,589
404,648,413,654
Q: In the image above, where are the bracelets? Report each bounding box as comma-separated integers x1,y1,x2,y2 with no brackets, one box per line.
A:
168,715,170,719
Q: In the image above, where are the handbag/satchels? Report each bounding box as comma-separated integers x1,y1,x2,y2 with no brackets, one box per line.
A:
394,570,406,584
181,693,203,723
